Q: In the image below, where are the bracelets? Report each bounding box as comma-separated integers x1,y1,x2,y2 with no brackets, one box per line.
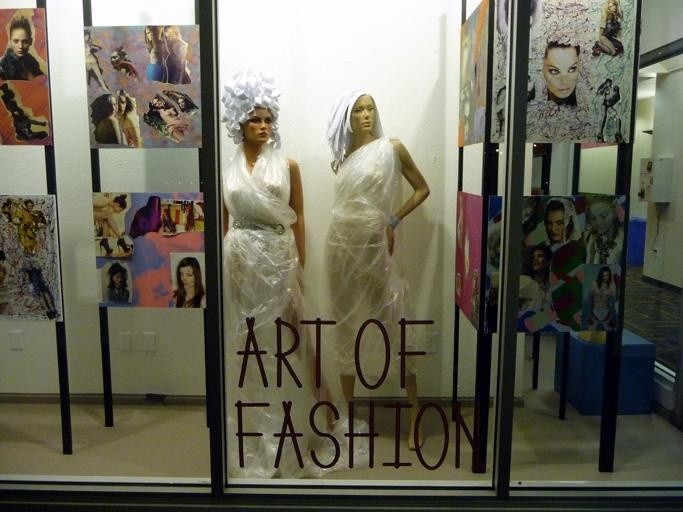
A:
388,215,399,228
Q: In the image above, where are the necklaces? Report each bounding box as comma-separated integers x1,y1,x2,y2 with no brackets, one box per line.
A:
244,142,262,164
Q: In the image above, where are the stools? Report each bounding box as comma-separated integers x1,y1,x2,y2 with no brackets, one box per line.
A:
554,328,657,416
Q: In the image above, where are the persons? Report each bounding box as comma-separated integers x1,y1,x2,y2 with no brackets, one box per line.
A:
220,71,338,442
591,0,625,56
106,262,132,303
16,259,60,320
172,257,206,308
330,90,429,450
526,42,600,142
521,197,623,329
92,193,133,256
0,16,46,83
90,26,198,148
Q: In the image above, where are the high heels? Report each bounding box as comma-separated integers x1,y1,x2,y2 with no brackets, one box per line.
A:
116,238,131,253
99,238,113,254
